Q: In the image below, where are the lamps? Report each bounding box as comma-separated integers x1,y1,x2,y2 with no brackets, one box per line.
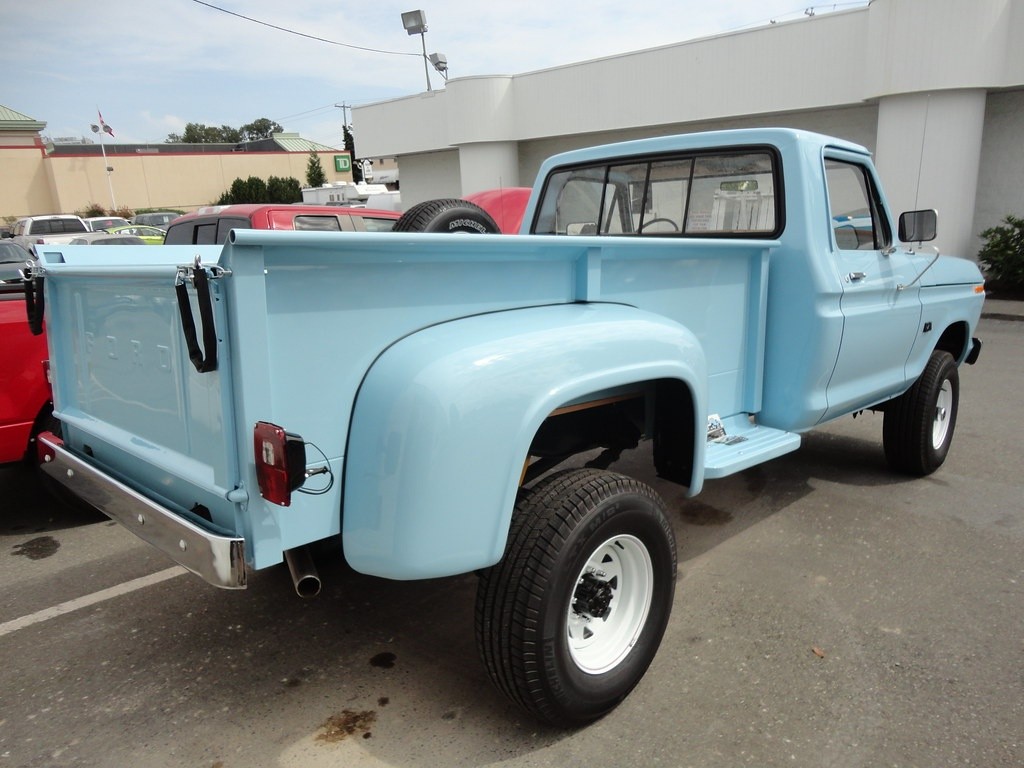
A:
401,9,432,91
429,53,448,81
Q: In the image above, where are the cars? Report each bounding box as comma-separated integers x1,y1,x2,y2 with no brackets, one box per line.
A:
0,187,533,507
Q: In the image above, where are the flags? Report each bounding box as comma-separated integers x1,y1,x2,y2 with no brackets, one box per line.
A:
99,110,116,137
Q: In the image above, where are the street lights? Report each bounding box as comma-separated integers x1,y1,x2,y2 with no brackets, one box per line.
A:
91,125,117,212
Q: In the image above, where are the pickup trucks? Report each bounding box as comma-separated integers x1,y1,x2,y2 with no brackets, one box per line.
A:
20,127,986,728
